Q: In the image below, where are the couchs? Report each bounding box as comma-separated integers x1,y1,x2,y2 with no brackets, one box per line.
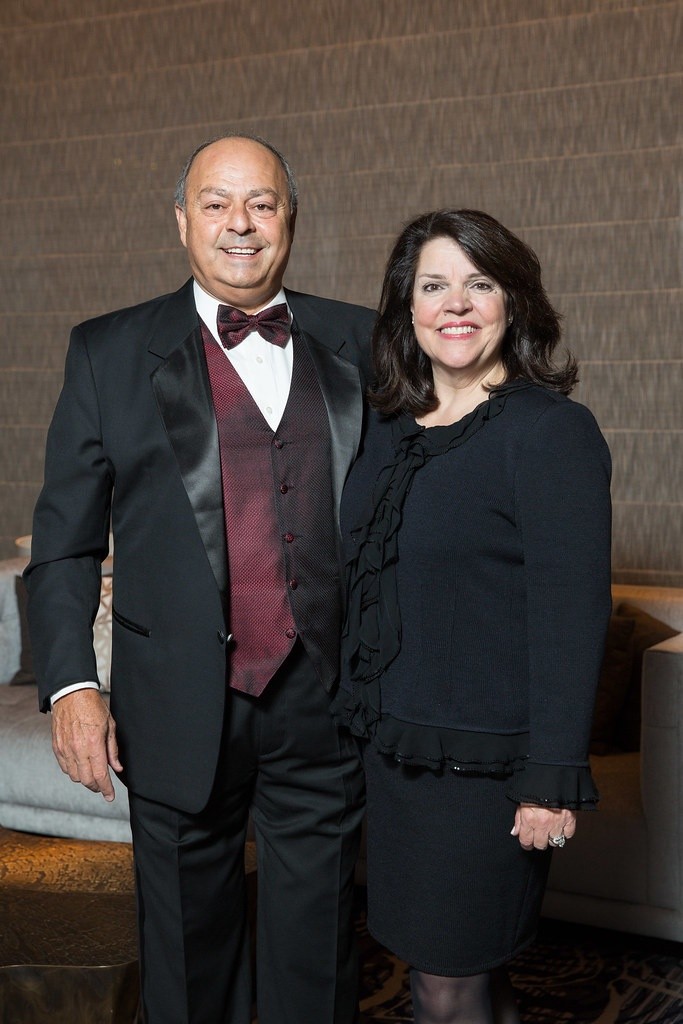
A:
0,555,683,962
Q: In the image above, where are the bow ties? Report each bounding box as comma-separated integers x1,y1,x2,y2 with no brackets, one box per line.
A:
216,303,290,350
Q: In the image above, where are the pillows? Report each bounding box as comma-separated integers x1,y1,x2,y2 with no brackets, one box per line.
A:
93,577,115,693
587,602,681,757
9,575,39,685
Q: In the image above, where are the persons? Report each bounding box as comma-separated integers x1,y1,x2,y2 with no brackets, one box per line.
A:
340,209,615,1024
22,139,381,1024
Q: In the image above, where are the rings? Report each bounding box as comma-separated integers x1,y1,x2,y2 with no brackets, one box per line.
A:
549,834,566,848
